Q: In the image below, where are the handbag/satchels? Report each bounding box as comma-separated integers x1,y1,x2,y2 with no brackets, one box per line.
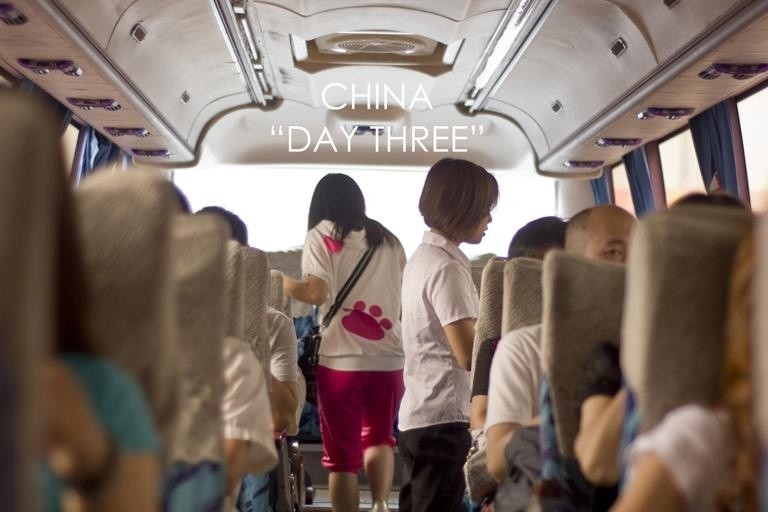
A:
297,334,321,405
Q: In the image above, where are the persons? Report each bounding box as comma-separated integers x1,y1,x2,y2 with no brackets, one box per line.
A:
605,211,767,512
193,203,307,441
397,155,499,512
570,191,745,510
162,179,281,512
38,133,166,512
281,173,407,512
468,215,565,433
482,203,640,512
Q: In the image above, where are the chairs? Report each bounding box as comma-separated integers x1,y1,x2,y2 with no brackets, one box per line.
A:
1,91,316,512
468,204,768,511
